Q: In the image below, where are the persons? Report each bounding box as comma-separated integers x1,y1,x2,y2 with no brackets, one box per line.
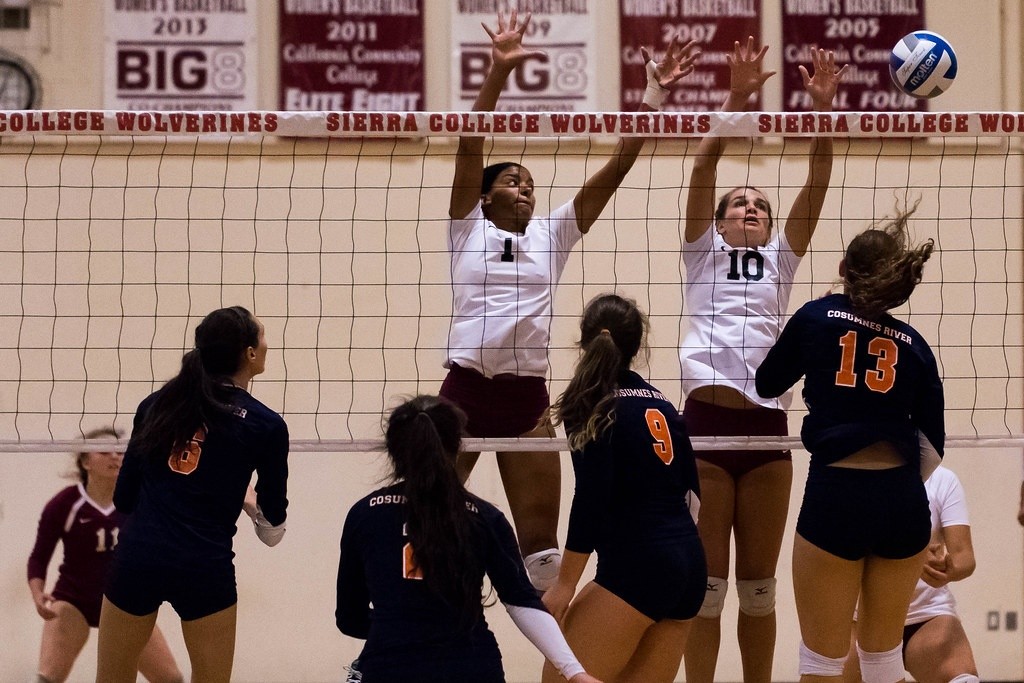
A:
541,294,707,683
96,306,289,683
435,9,700,605
680,37,980,683
324,394,595,683
27,427,185,683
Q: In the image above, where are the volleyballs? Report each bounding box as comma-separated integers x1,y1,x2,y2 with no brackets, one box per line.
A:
889,29,959,99
